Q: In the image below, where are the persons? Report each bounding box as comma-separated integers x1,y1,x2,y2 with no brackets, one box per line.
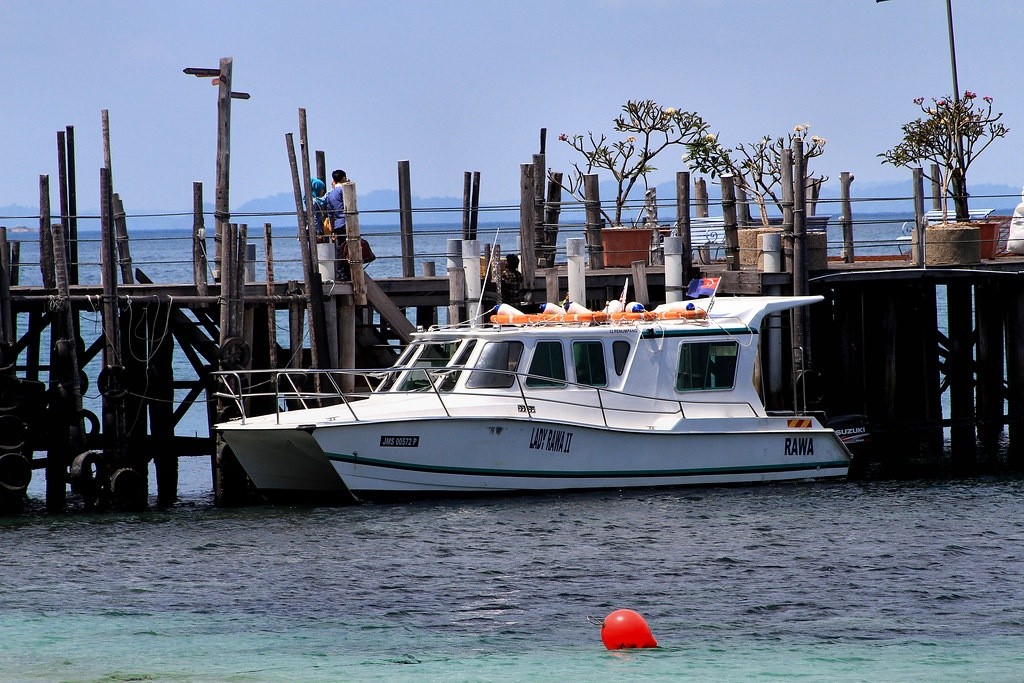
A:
303,177,329,244
502,254,524,309
326,170,348,252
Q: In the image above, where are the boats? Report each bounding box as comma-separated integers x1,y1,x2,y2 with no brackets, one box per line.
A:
209,226,856,504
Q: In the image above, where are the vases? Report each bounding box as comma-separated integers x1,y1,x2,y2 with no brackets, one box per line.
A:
600,222,1000,271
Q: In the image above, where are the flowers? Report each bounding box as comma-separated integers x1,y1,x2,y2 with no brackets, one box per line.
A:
547,91,1011,231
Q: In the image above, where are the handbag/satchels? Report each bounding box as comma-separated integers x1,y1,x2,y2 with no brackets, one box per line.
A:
323,218,331,235
341,238,376,271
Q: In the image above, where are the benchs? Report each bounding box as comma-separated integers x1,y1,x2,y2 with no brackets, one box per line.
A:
652,216,728,266
897,208,994,256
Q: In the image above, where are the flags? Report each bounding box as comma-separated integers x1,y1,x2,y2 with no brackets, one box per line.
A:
618,286,626,303
686,278,719,298
